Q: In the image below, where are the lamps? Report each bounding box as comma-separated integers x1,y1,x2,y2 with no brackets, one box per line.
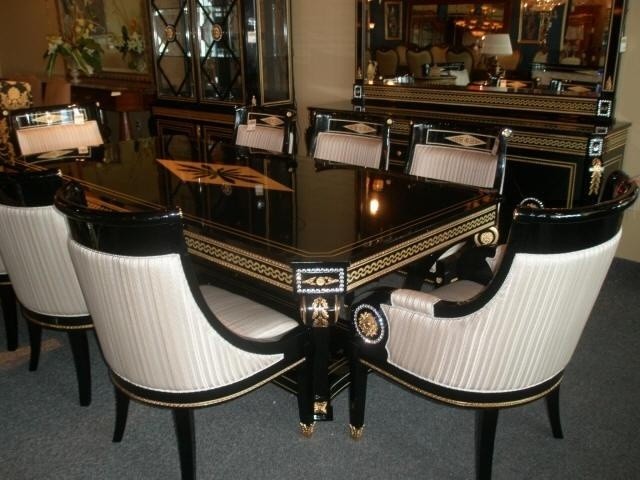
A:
395,43,412,69
480,34,511,92
498,48,520,72
374,49,398,78
430,43,449,65
445,48,472,78
406,50,433,78
519,0,563,44
368,22,376,30
454,4,504,40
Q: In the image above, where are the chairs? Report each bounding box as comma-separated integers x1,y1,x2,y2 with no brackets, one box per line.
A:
1,167,95,408
58,180,314,479
234,107,295,158
406,119,511,196
310,108,389,168
348,169,640,480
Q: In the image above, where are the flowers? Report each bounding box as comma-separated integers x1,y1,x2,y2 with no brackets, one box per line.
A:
113,18,148,76
41,1,109,81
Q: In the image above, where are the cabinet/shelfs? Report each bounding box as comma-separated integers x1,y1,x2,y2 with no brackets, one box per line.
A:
143,1,291,117
69,71,151,141
352,104,631,240
153,118,236,165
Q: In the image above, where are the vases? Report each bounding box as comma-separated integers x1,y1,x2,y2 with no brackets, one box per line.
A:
134,59,147,73
63,59,82,88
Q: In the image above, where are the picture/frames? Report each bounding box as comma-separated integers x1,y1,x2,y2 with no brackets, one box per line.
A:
517,0,544,45
383,1,403,41
556,1,615,72
355,0,619,118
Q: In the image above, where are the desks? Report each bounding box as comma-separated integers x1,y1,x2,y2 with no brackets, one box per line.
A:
13,130,499,421
